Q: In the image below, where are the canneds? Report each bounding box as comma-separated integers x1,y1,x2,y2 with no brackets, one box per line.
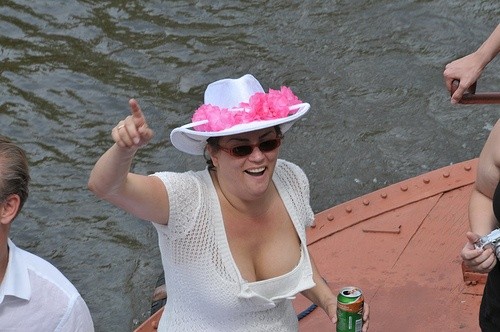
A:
336,287,365,332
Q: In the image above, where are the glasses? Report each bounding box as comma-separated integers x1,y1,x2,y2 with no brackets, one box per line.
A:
216,135,284,157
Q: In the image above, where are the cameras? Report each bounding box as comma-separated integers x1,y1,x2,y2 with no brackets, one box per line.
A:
474,228,500,261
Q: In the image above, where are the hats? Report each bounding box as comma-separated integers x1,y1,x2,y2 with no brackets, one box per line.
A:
170,74,311,155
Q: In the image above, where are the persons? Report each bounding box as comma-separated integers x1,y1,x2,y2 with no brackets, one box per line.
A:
443,23,500,105
461,118,500,332
0,142,95,332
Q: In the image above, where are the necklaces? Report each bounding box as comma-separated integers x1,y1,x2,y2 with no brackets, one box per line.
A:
88,74,369,332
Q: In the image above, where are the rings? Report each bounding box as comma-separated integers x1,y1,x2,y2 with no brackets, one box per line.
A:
118,125,125,130
